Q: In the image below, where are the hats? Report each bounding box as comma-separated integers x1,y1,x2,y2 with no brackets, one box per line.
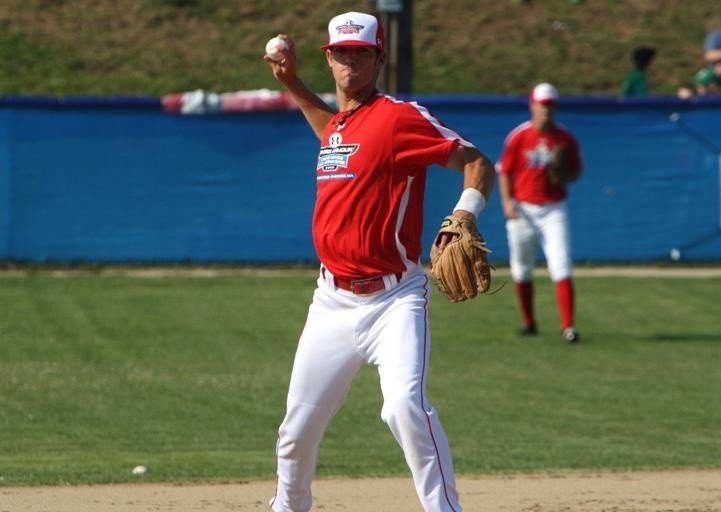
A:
530,82,558,104
319,11,384,51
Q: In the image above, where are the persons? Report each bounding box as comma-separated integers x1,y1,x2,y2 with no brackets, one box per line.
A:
620,28,721,100
263,12,494,512
494,83,580,342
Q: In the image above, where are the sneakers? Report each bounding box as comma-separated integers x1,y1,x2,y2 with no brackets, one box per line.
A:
560,329,580,341
518,325,536,336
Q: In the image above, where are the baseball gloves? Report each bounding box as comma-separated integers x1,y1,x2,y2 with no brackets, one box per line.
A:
430,214,506,302
547,143,573,187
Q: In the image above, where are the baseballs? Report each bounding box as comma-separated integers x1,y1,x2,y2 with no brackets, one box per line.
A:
266,36,288,60
133,465,147,474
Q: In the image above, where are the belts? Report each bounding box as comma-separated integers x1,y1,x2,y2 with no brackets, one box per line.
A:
321,267,401,294
